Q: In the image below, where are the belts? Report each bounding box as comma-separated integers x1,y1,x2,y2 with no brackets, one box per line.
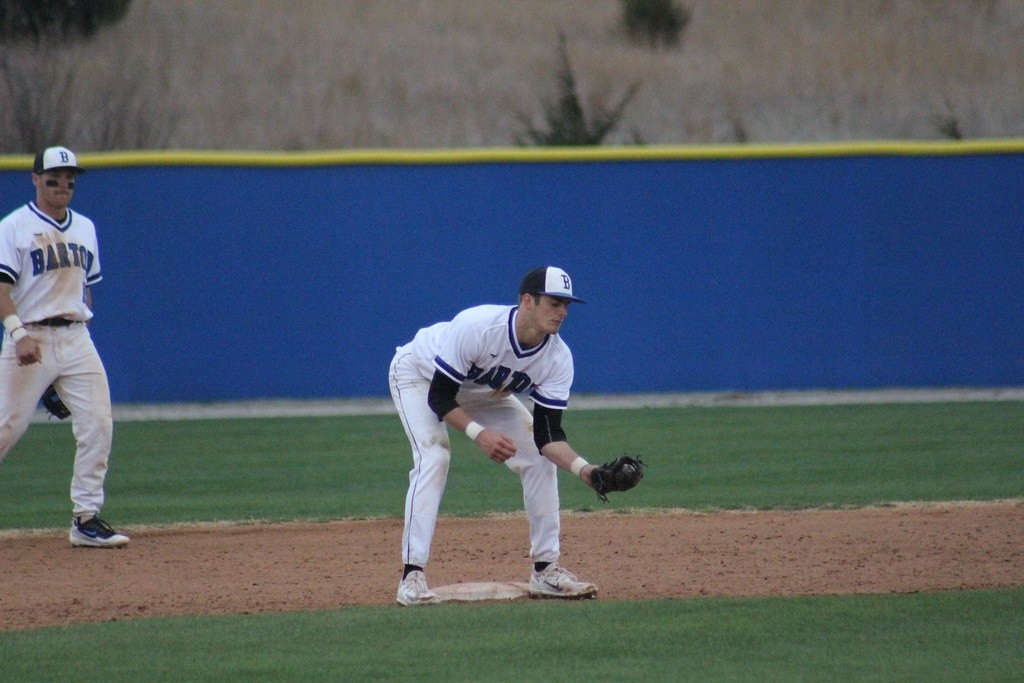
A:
37,317,82,326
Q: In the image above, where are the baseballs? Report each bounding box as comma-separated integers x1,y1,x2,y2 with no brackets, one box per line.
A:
622,463,635,475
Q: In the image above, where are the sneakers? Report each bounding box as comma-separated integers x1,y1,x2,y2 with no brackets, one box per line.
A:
395,570,441,607
528,562,598,599
68,514,131,549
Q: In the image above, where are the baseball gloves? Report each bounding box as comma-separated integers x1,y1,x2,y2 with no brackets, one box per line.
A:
42,384,71,420
588,455,644,494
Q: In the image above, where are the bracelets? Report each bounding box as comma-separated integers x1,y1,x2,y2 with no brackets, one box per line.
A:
571,457,589,477
465,422,485,441
11,328,28,343
3,314,23,335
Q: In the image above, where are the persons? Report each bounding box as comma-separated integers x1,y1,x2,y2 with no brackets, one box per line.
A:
389,266,644,607
0,146,129,548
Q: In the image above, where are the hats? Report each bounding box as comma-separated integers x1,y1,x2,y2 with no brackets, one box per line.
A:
32,145,86,175
520,266,590,304
